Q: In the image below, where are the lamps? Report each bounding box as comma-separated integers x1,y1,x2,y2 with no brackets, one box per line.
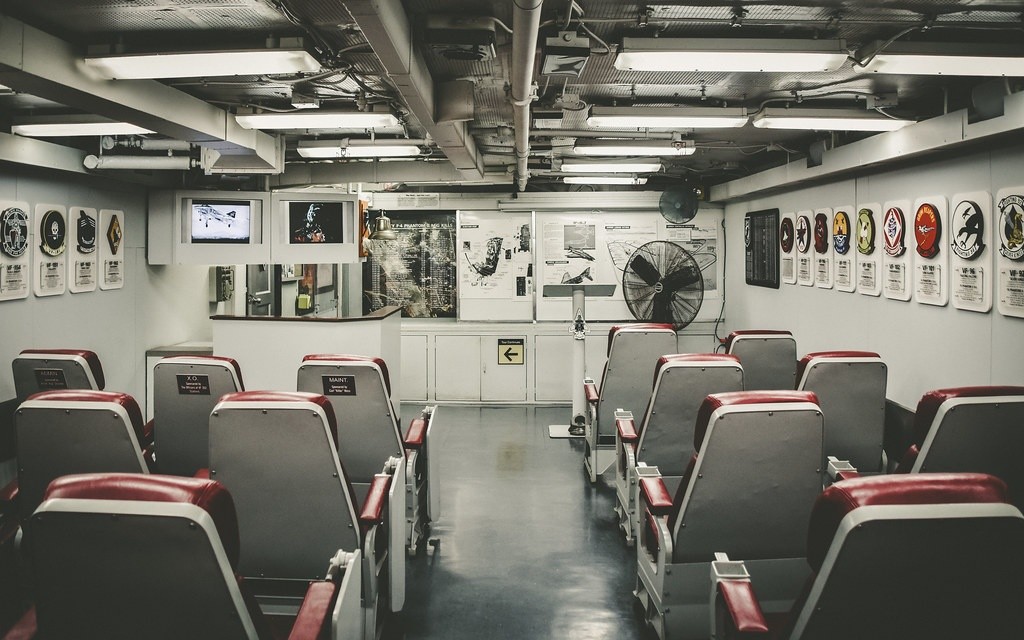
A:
560,37,1024,187
12,50,421,157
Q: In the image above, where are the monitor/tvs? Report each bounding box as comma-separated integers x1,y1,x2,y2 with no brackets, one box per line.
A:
181,200,261,244
280,201,354,245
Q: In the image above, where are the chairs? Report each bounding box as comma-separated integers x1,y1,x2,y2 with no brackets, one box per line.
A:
0,349,437,640
582,325,1024,640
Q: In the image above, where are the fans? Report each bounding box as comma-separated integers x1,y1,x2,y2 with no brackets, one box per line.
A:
659,185,699,224
622,240,704,331
